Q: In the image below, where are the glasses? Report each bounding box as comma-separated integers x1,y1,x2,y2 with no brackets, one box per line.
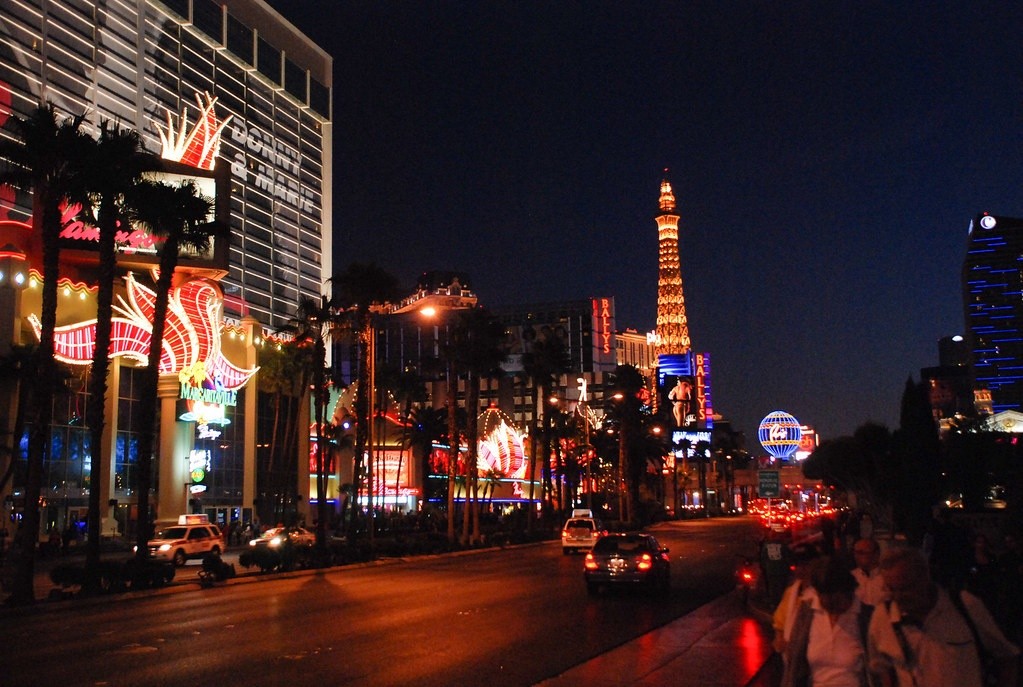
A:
854,550,873,558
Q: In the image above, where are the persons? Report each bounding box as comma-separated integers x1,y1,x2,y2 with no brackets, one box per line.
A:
771,429,1023,687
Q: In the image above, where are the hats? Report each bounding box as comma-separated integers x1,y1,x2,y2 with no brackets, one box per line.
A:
785,543,820,562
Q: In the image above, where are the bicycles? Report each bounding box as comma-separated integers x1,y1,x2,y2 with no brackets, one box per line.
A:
734,551,762,608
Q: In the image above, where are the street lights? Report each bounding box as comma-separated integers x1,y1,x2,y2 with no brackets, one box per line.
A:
366,305,437,556
582,393,626,515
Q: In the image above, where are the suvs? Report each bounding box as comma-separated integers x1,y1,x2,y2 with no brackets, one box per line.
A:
133,525,225,569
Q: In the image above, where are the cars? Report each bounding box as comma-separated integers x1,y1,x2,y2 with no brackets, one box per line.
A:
249,527,316,550
560,516,609,556
582,533,672,598
